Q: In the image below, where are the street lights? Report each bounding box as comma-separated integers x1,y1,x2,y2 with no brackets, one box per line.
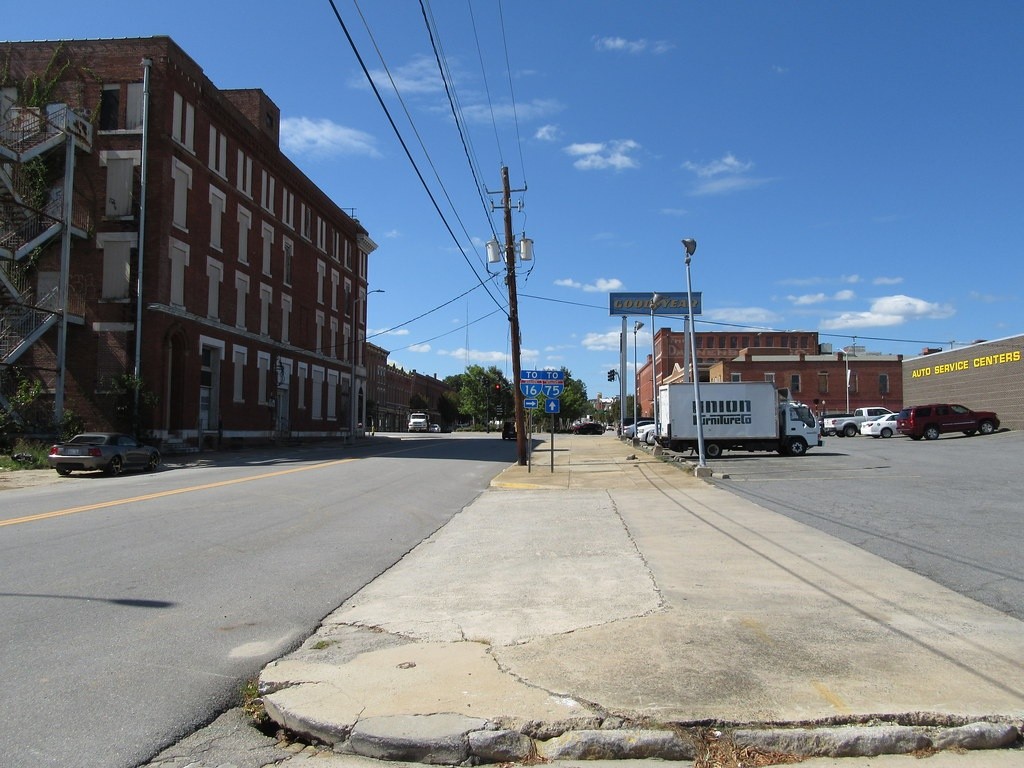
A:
632,320,645,438
681,236,707,469
607,369,621,438
648,291,662,439
484,165,534,468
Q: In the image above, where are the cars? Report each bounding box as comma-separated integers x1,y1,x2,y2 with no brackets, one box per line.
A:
573,422,605,436
637,424,659,442
647,427,660,444
50,431,163,476
606,424,614,431
429,423,441,433
859,413,901,439
624,420,654,440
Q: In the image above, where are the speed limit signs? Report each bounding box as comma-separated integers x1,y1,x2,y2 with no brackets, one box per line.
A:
519,370,543,398
542,371,564,399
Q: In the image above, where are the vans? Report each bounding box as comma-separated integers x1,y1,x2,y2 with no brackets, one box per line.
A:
616,417,655,437
817,413,854,437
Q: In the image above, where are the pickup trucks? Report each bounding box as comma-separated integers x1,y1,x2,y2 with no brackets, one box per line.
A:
824,407,893,437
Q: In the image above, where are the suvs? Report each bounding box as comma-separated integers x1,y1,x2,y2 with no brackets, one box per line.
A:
896,404,1000,440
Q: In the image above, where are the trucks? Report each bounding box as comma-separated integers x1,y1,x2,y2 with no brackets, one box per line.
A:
408,409,430,433
659,381,823,460
